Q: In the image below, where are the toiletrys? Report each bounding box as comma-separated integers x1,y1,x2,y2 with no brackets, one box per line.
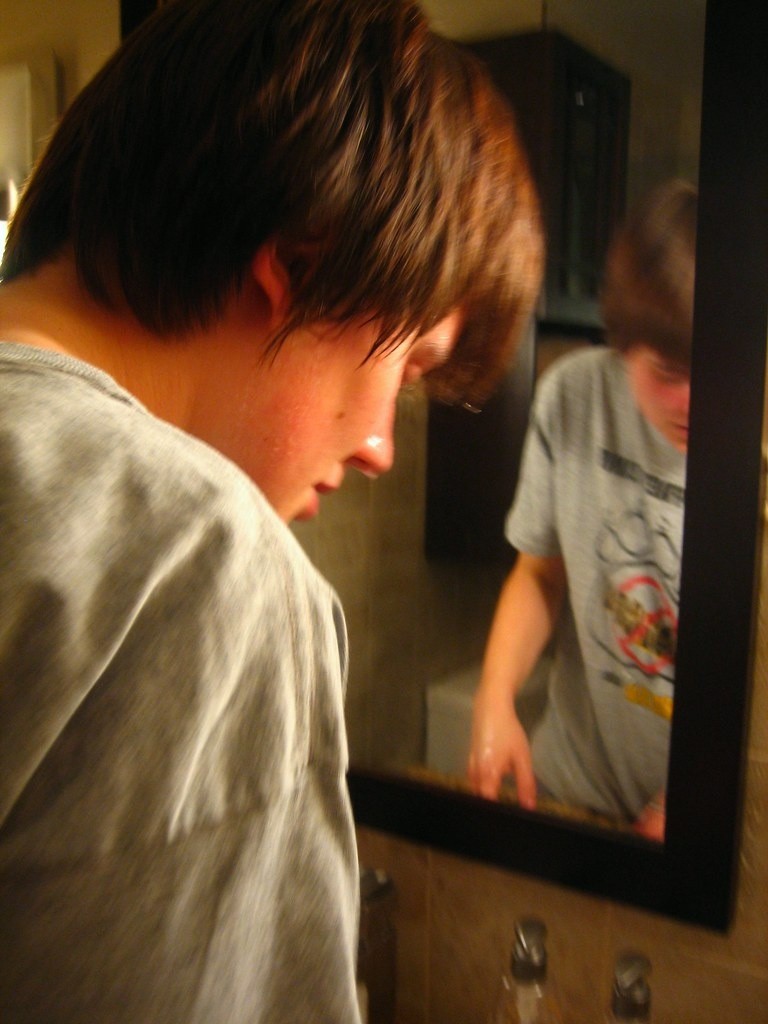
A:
608,947,652,1024
489,916,550,1024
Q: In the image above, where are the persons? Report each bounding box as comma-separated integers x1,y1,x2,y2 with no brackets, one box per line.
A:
0,0,545,1024
466,134,701,841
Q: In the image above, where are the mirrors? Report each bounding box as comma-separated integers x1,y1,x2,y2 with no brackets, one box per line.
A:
117,0,768,937
